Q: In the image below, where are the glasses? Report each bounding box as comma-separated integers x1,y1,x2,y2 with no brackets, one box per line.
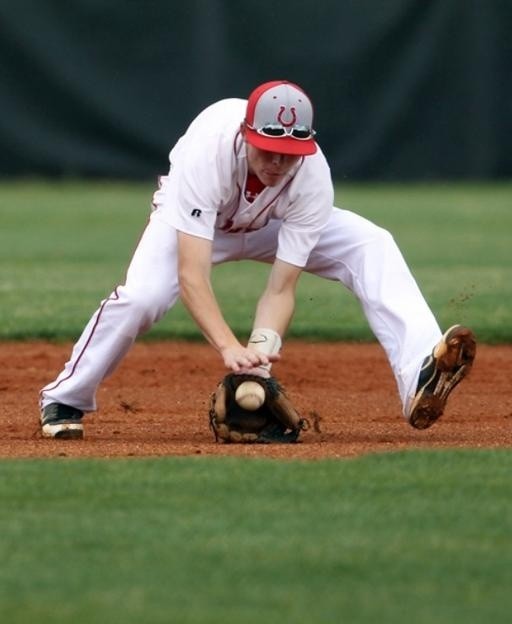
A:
246,123,314,140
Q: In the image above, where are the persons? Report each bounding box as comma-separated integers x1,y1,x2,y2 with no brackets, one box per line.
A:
39,81,477,443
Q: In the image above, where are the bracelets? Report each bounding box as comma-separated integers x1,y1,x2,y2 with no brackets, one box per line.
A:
246,327,282,371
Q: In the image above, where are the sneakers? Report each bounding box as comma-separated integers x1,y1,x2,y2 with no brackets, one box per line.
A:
40,403,85,440
402,323,476,429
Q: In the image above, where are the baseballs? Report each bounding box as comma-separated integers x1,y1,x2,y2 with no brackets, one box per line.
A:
234,381,265,410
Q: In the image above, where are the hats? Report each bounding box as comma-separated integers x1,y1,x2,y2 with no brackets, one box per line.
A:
244,80,317,157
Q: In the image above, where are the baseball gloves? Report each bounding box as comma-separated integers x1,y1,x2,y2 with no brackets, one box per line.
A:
207,368,309,444
